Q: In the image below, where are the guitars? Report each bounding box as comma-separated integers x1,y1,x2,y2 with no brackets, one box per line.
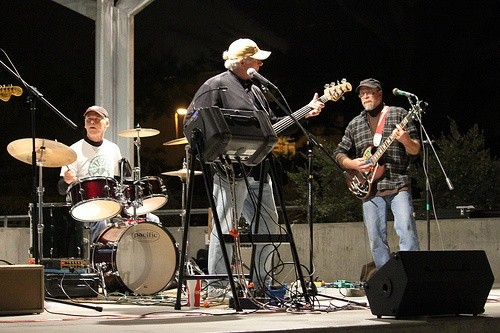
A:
343,99,429,200
0,84,23,102
211,78,352,182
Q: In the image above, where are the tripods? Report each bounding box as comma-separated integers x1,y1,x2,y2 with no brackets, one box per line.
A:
261,86,367,306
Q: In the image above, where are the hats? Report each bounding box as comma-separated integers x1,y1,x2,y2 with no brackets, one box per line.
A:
228,38,272,60
356,78,382,90
84,106,109,118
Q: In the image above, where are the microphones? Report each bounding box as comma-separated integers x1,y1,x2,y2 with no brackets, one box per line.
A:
247,68,278,91
392,88,416,98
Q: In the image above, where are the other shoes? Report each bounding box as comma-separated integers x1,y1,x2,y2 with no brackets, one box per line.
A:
208,286,229,298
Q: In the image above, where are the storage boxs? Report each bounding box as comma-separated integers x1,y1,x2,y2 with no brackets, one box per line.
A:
44,268,100,298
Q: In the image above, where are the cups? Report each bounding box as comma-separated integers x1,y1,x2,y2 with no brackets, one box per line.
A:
186,279,200,308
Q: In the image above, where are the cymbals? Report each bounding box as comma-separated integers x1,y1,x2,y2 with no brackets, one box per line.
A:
162,137,188,146
116,129,161,137
7,138,77,167
161,170,203,178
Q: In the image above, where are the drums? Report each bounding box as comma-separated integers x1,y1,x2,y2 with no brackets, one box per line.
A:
87,217,180,297
65,176,124,223
122,176,168,216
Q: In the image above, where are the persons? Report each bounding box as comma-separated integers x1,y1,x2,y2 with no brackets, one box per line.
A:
332,78,422,271
57,105,122,242
182,38,325,302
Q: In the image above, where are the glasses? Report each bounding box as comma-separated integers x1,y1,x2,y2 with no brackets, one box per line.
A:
359,89,378,97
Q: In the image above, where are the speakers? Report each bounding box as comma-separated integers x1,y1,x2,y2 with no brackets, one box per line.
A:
0,264,44,316
363,249,495,320
233,234,300,289
